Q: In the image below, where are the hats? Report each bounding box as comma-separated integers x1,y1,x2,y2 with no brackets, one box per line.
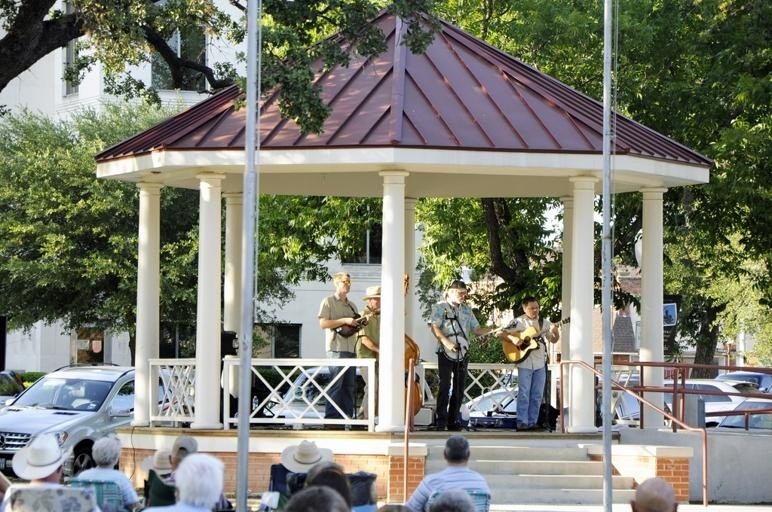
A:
12,433,72,481
280,440,333,473
448,280,466,289
141,448,175,475
170,437,198,460
361,286,380,300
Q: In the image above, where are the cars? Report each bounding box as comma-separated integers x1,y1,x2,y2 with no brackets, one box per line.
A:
460,385,640,429
717,397,772,434
0,370,24,407
660,379,745,427
712,372,771,394
618,374,639,384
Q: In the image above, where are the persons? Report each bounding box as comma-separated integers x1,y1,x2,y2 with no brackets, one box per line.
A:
404,433,492,511
495,294,559,431
631,477,678,512
317,272,369,429
427,279,503,431
349,285,381,430
1,433,410,510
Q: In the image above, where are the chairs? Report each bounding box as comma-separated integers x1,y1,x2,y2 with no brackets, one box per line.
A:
0,464,377,512
424,491,491,512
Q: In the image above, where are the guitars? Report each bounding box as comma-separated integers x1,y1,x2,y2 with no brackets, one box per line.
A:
335,309,380,337
502,316,570,363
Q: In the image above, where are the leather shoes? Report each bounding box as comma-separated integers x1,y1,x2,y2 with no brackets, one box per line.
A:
515,426,530,432
529,425,545,432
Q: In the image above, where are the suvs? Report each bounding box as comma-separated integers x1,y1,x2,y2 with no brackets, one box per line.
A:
268,366,366,430
0,363,194,478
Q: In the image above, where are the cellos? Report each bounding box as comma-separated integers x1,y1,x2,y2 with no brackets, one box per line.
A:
375,272,423,417
